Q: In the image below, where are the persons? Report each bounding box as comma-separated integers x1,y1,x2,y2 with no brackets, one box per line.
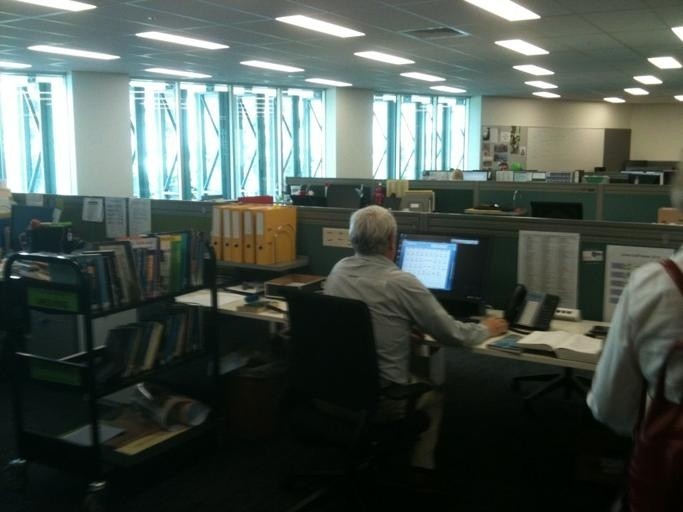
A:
323,206,509,489
499,163,507,171
452,169,462,180
586,145,683,511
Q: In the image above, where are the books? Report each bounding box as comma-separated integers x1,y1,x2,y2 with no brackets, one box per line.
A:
486,333,528,355
105,305,204,379
1,227,204,310
61,393,209,454
237,302,279,312
516,328,604,365
382,179,436,212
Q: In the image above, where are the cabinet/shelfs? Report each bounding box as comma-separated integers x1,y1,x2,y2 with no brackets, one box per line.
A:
3,241,218,494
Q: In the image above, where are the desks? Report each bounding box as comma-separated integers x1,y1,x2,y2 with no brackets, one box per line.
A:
173,288,606,370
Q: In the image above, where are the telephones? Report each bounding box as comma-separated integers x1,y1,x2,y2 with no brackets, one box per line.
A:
504,284,559,330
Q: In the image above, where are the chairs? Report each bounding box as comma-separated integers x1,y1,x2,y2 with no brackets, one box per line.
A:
276,289,438,512
507,366,596,407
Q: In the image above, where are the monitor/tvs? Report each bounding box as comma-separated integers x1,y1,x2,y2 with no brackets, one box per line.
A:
9,205,52,250
462,170,488,181
609,177,628,184
529,200,584,220
627,171,664,185
594,167,606,171
394,230,461,297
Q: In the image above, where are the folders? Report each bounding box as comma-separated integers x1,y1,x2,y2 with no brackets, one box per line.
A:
211,203,296,266
